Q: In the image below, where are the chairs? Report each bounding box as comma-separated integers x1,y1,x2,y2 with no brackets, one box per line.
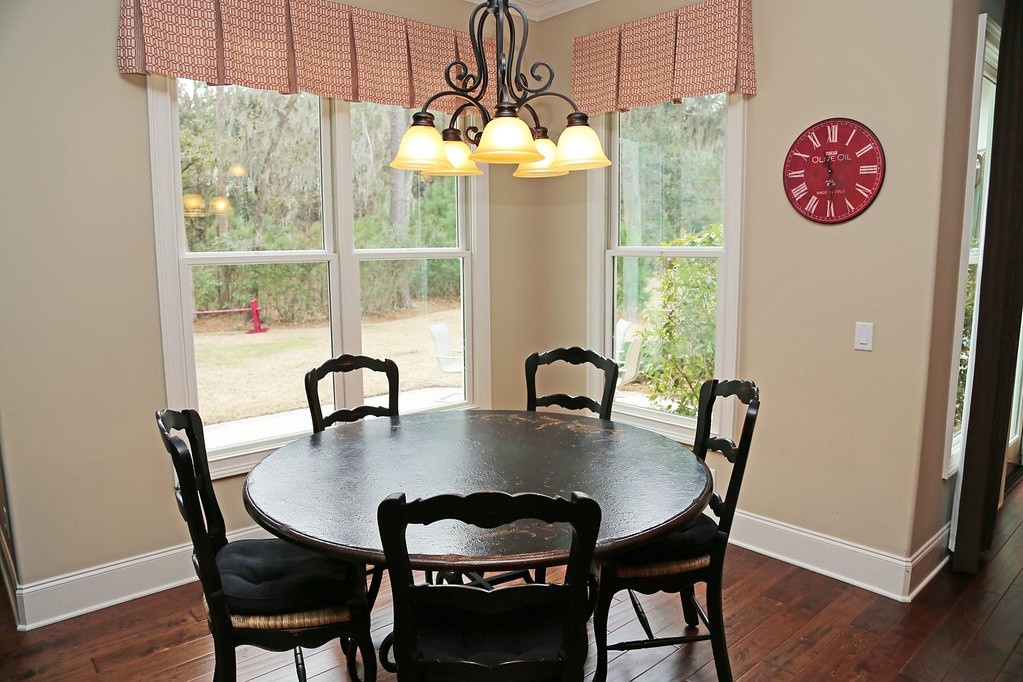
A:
376,490,602,682
305,353,400,434
615,338,645,388
615,317,630,398
525,346,618,421
428,321,463,402
154,407,378,682
589,379,760,682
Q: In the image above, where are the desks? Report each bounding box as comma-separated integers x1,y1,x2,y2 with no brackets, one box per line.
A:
242,410,714,682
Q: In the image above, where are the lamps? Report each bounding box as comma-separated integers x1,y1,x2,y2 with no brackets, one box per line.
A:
183,151,253,219
389,0,613,177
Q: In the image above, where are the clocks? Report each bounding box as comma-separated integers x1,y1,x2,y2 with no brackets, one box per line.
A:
783,117,887,225
